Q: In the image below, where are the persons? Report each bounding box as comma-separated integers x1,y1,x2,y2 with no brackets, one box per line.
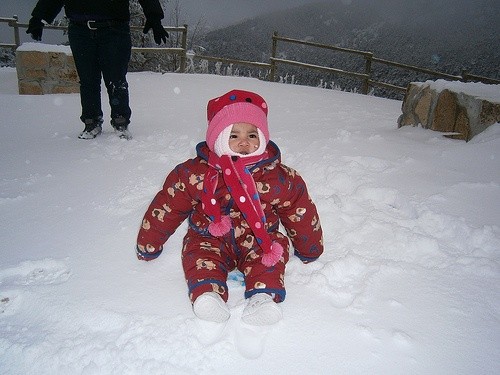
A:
135,89,324,326
26,0,169,140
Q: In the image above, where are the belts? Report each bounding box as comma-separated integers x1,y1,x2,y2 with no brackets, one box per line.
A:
70,18,125,30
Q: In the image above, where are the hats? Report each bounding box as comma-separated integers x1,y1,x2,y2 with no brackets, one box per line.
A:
204,88,271,153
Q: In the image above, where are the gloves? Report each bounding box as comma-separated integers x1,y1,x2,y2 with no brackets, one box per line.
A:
26,14,44,42
142,18,170,45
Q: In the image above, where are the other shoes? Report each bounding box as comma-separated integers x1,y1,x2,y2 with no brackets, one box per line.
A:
78,121,103,141
113,124,132,141
241,293,282,326
193,291,231,323
